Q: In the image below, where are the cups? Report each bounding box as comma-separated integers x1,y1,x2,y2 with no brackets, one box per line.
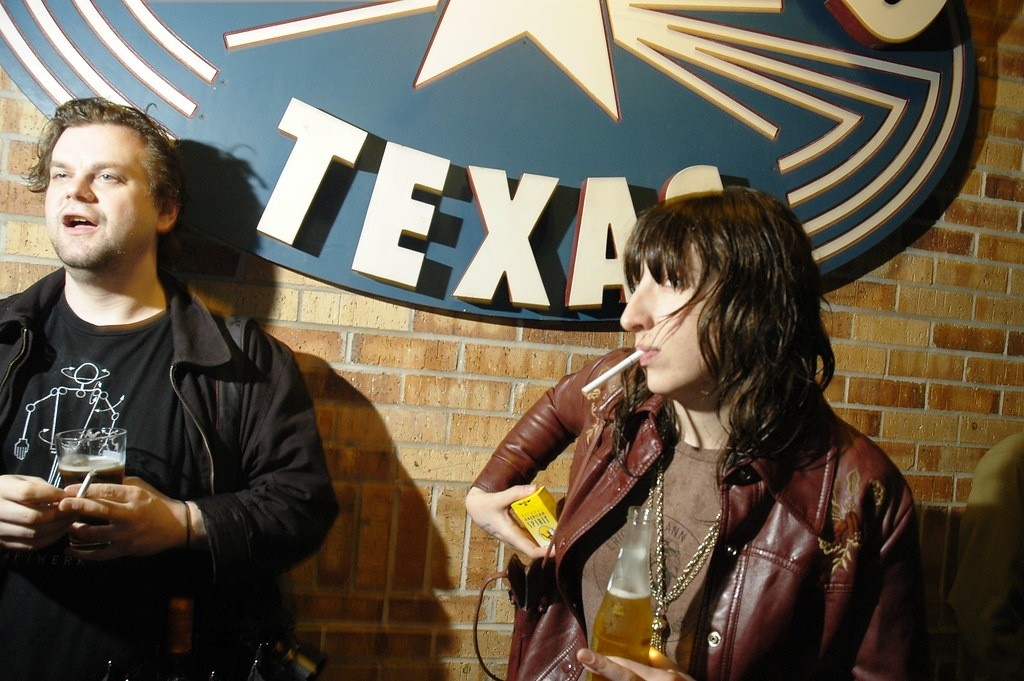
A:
55,427,127,551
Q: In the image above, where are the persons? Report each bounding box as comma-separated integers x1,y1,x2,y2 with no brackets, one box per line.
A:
0,94,340,681
948,431,1024,681
463,185,933,681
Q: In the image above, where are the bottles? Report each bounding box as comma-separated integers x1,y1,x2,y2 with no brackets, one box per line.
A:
584,506,655,681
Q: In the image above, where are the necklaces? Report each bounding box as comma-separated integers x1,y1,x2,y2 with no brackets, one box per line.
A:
645,395,725,654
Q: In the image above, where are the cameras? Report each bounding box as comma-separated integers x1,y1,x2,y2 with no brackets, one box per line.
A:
240,611,328,681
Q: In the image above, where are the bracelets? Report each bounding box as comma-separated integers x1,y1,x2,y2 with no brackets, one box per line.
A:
181,501,191,551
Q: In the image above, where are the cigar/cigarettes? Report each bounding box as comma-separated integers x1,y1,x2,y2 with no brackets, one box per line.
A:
581,350,645,394
77,469,97,499
